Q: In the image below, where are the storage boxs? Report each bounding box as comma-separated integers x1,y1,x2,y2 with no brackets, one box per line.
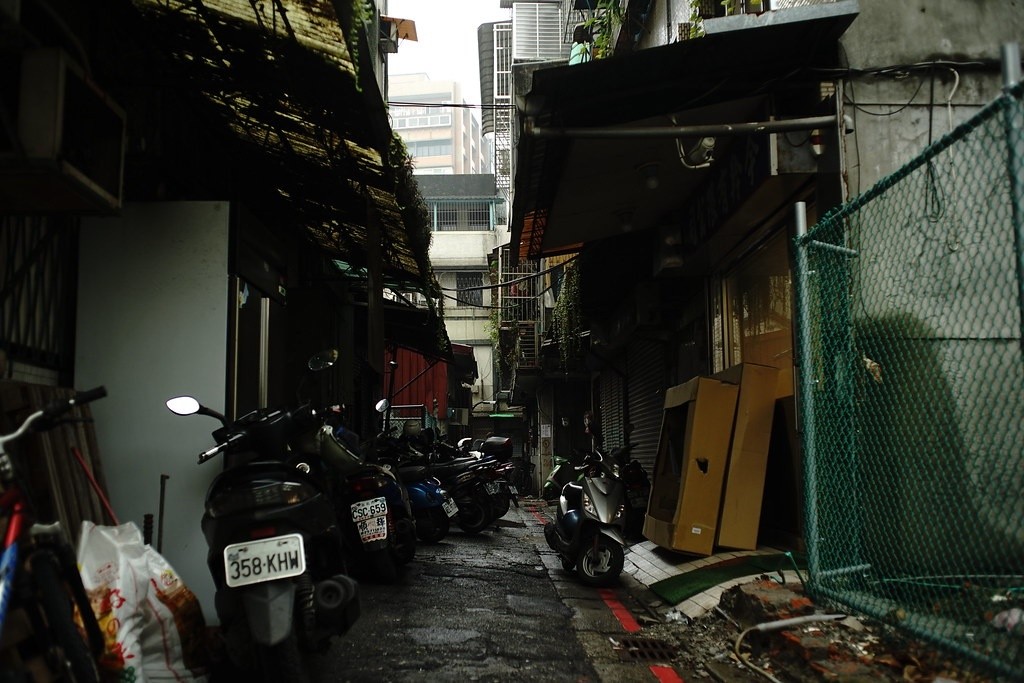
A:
709,361,781,550
640,375,740,555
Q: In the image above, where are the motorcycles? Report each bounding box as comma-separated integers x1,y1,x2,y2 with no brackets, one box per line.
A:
544,421,628,588
164,346,363,670
321,394,652,574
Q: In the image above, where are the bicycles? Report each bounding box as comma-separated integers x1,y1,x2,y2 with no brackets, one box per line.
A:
0,383,135,683
